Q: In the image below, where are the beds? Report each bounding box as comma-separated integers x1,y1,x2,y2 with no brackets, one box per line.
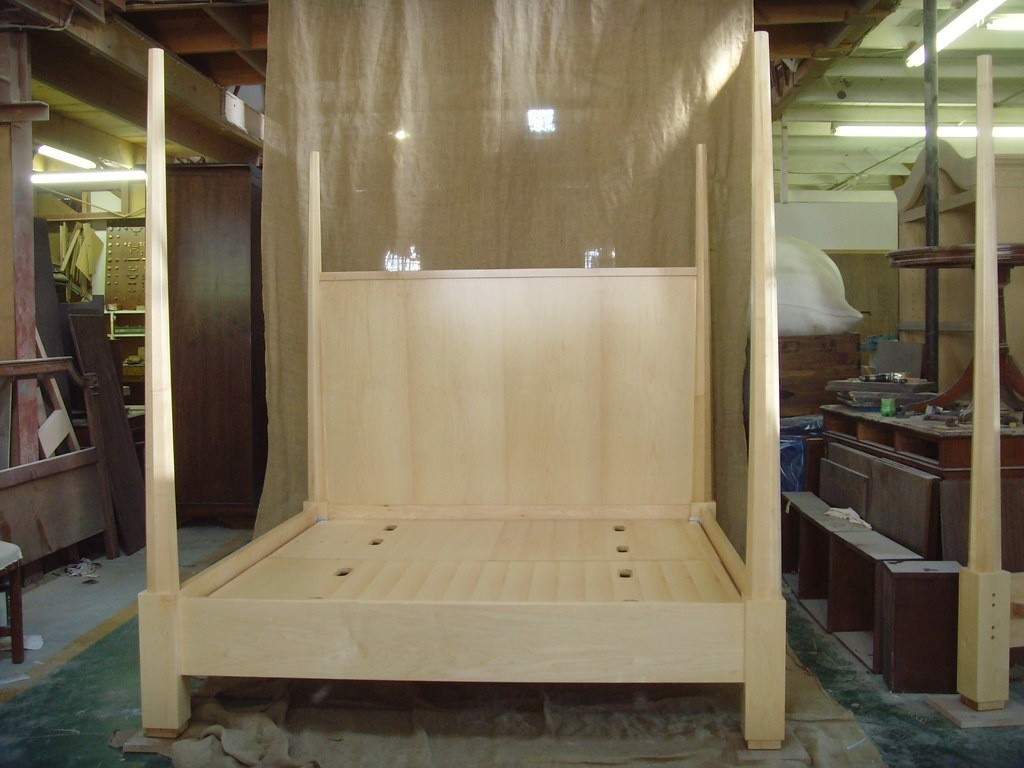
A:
136,30,785,751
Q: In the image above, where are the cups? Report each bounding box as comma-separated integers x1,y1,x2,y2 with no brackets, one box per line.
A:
881,398,895,416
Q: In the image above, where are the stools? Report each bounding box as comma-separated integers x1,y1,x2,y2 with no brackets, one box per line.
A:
0,540,25,664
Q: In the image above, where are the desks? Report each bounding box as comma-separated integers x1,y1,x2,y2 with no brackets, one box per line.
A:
819,404,1024,561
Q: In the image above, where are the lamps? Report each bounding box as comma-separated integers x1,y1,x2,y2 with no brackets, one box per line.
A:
830,122,978,138
904,0,1006,68
977,14,1024,31
